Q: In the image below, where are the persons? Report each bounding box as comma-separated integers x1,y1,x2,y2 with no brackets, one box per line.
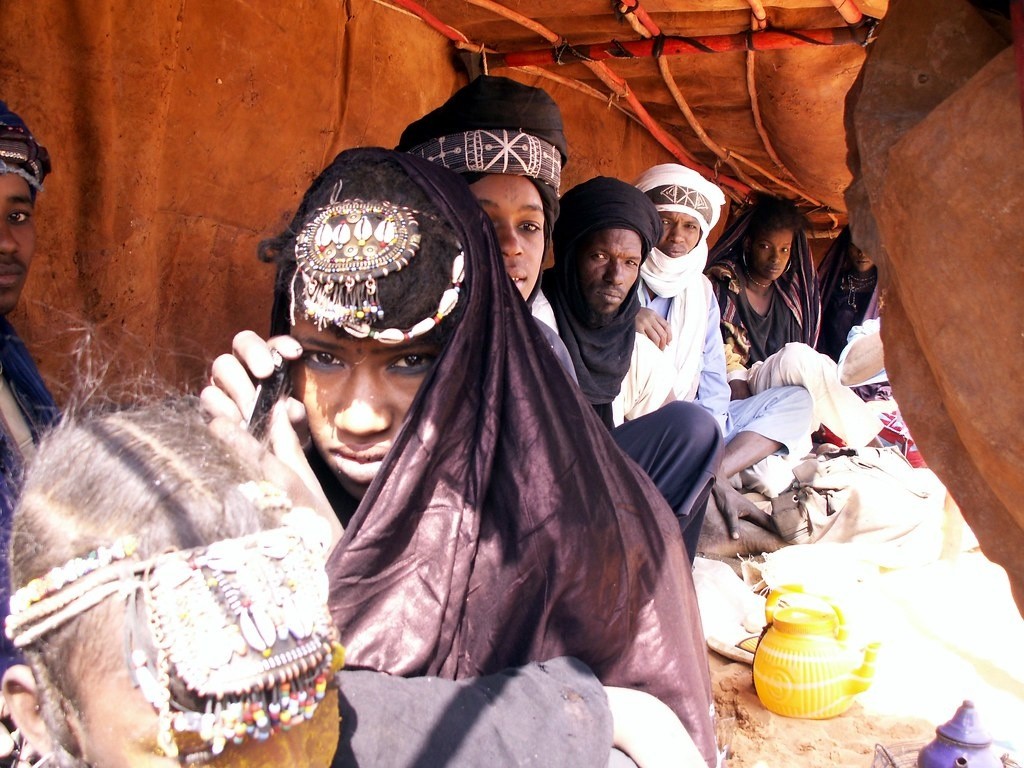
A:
0,74,929,768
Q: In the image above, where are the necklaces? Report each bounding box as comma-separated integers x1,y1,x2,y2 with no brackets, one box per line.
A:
847,274,876,309
746,271,772,288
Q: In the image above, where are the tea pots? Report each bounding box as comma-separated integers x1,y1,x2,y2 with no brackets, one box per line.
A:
917,700,1003,768
754,584,880,719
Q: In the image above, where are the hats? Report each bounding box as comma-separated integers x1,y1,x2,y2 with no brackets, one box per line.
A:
394,77,566,199
632,163,726,256
550,174,663,269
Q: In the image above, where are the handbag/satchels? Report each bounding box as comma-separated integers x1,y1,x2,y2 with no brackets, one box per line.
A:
766,447,914,545
